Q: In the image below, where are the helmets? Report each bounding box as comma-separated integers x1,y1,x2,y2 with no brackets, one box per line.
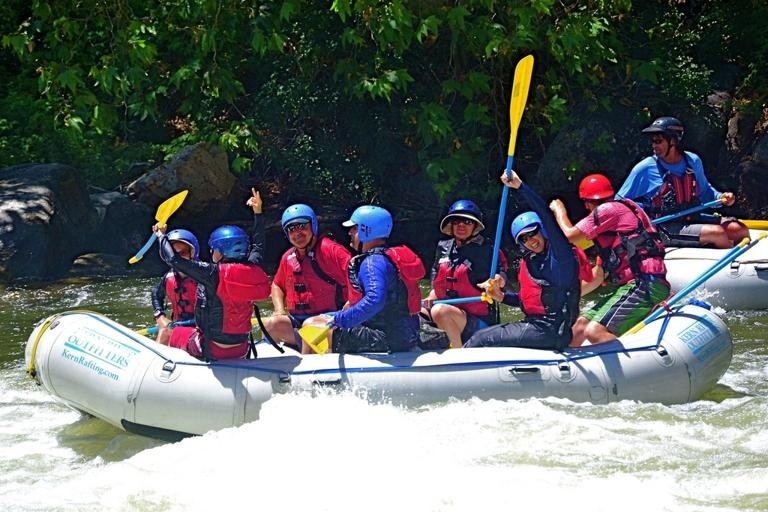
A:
207,225,251,262
578,174,614,201
165,229,201,262
281,204,319,238
343,205,393,243
510,210,548,245
642,117,685,145
448,199,482,222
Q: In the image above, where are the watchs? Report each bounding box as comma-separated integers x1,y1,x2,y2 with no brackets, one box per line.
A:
152,310,166,320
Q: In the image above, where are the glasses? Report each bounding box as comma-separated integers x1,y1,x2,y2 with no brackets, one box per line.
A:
451,218,475,225
520,228,540,244
287,222,310,231
650,138,664,144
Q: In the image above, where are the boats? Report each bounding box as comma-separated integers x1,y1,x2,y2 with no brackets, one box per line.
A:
23,296,734,446
662,217,768,311
25,303,734,436
625,220,768,309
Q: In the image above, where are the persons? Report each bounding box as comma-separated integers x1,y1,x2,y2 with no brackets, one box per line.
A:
152,188,271,361
461,168,593,348
614,117,751,249
262,203,362,348
301,205,426,354
550,174,671,347
151,229,198,348
420,200,508,348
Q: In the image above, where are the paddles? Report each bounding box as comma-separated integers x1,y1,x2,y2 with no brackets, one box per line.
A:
297,323,334,355
576,198,728,249
489,54,535,284
128,189,189,263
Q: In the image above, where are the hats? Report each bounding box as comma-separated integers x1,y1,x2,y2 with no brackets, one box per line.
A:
439,213,485,237
